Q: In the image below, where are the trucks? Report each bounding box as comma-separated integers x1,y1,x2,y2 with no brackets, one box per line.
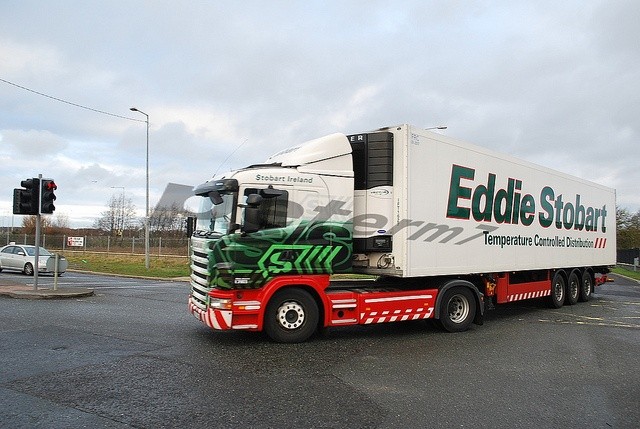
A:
184,123,617,345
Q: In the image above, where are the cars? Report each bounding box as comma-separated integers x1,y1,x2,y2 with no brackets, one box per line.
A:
0,244,66,276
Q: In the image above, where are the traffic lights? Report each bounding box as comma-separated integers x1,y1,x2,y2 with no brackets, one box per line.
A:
19,178,38,215
184,215,195,238
41,179,57,214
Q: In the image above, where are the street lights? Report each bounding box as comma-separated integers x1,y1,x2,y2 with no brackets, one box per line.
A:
426,125,448,131
130,108,152,270
